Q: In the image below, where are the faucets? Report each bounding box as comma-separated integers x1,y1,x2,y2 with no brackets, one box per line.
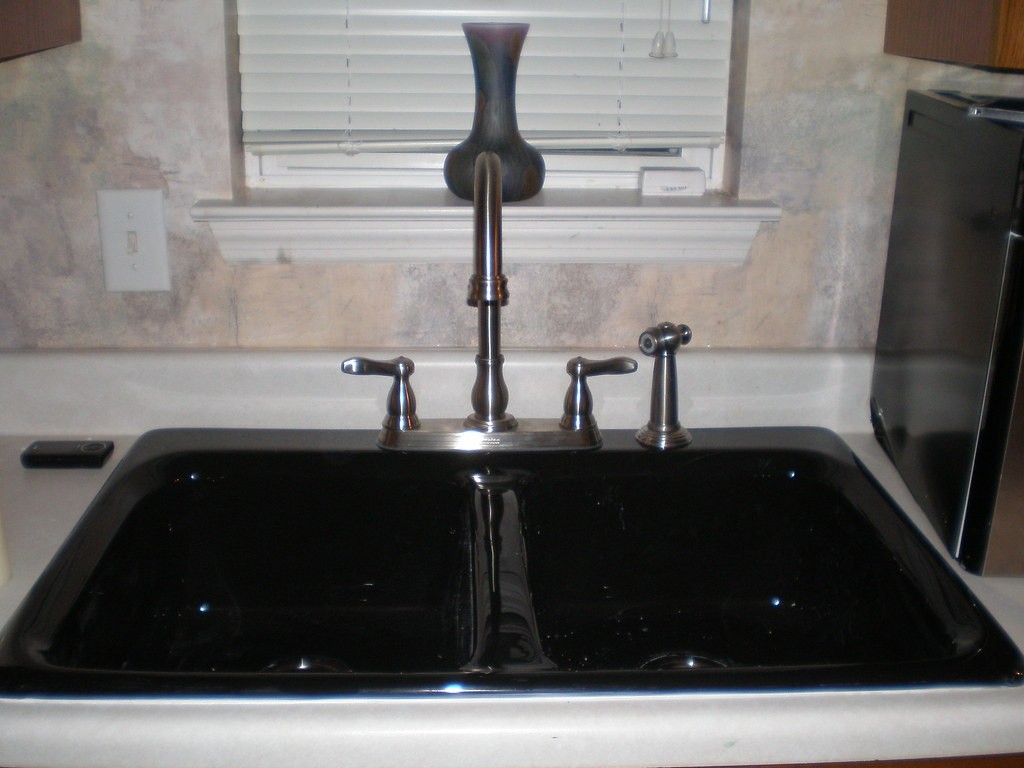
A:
460,151,512,429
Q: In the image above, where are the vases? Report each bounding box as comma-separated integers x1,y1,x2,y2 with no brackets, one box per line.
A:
444,22,545,203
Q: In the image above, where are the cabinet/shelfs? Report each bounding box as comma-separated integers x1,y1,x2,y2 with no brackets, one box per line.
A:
882,0,1024,73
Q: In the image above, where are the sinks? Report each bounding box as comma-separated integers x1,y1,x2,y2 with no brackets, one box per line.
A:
0,417,490,699
507,418,1023,695
